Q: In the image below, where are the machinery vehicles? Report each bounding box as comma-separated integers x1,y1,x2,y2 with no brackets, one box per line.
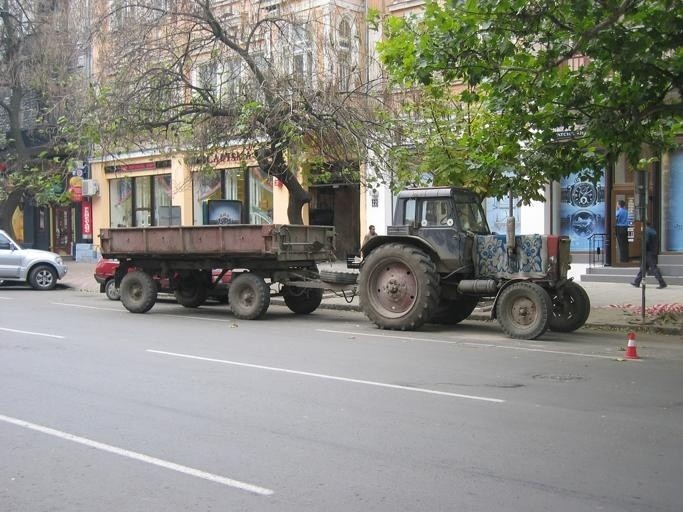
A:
343,173,593,345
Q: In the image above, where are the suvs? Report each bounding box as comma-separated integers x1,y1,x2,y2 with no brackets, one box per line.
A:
0,227,69,293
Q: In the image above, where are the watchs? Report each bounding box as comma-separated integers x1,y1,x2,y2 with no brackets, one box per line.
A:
560,208,605,238
560,181,605,209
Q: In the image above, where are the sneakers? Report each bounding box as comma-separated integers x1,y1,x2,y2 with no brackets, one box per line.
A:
656,283,667,289
630,280,641,289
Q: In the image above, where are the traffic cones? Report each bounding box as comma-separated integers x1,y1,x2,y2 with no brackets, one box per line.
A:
622,330,640,360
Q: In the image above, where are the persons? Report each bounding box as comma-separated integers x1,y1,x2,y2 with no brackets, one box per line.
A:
615,200,629,262
362,225,378,248
630,220,667,289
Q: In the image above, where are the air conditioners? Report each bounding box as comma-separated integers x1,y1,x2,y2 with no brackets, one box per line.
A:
81,180,98,198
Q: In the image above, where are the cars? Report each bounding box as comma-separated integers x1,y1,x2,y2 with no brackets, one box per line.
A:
91,247,233,308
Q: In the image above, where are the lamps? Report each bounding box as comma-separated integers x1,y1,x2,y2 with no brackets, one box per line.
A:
370,188,379,196
